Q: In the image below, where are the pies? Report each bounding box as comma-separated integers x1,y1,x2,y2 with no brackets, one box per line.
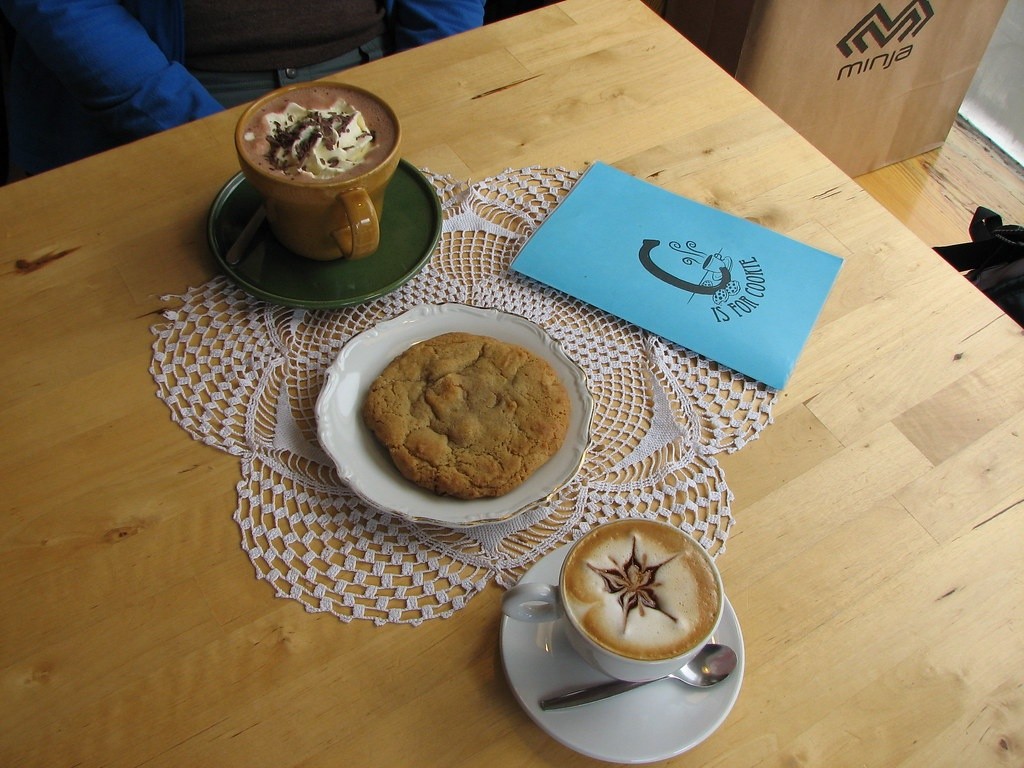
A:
362,327,570,497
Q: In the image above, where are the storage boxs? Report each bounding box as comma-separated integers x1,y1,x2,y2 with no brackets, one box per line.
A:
645,0,1009,180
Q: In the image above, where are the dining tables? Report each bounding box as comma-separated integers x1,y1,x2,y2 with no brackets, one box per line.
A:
2,1,1023,767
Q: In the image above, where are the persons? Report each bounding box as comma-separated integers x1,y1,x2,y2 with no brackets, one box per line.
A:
1,2,486,139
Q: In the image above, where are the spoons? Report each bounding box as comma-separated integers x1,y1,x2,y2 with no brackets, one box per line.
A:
540,644,737,711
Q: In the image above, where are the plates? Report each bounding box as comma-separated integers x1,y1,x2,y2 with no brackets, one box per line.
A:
207,158,443,309
500,542,747,765
313,302,594,529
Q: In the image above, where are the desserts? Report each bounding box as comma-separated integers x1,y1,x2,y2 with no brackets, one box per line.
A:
262,98,374,181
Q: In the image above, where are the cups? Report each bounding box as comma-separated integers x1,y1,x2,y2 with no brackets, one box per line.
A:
500,518,724,682
235,82,401,261
